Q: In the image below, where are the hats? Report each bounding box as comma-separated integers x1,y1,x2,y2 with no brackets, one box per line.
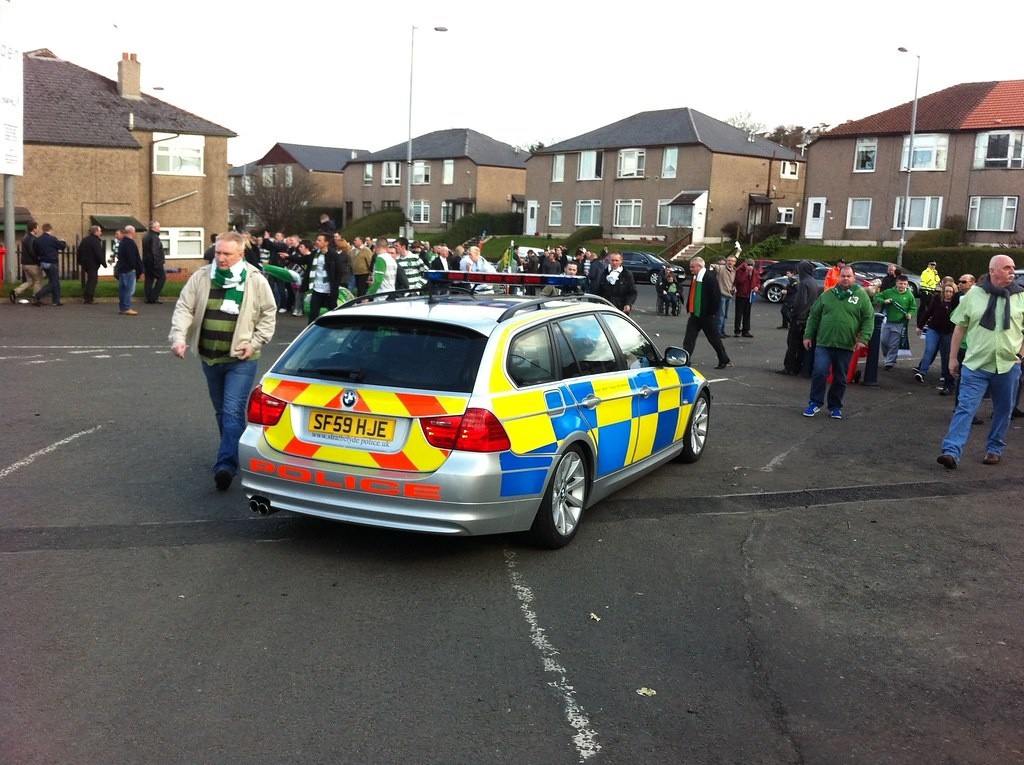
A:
745,258,755,265
839,258,846,263
928,260,937,264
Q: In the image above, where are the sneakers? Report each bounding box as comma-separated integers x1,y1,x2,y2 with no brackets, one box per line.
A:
803,402,821,417
829,408,842,419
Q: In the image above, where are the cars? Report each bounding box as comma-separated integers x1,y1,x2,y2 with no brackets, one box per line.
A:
620,251,686,285
516,246,544,260
238,270,713,549
846,261,921,298
735,260,779,275
758,267,881,303
758,259,827,287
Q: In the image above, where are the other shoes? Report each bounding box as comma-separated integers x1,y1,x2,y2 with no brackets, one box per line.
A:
777,325,788,329
52,302,64,306
939,386,951,395
936,454,957,469
122,309,137,314
972,416,984,424
9,290,17,303
912,366,920,372
734,331,754,338
719,332,731,337
28,295,42,307
671,310,676,315
279,308,287,313
214,468,232,491
991,407,1024,420
292,308,304,317
145,299,163,304
914,373,924,383
983,454,1000,465
714,359,730,369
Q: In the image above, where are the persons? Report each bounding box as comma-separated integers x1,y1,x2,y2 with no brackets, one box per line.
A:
10,221,67,306
169,232,278,490
710,247,760,338
683,257,730,369
510,244,638,316
656,265,682,316
937,254,1024,470
776,258,918,375
204,213,497,326
802,267,875,418
79,225,107,305
108,220,166,315
912,260,1024,425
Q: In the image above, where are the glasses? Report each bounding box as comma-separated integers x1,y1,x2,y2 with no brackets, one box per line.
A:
747,263,753,266
958,280,972,284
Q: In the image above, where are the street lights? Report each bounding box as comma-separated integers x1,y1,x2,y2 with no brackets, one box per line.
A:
897,46,921,267
404,26,447,239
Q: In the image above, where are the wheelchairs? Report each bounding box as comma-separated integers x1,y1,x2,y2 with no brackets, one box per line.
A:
656,294,682,316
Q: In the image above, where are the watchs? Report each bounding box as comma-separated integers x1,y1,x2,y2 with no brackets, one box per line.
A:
1016,354,1024,361
